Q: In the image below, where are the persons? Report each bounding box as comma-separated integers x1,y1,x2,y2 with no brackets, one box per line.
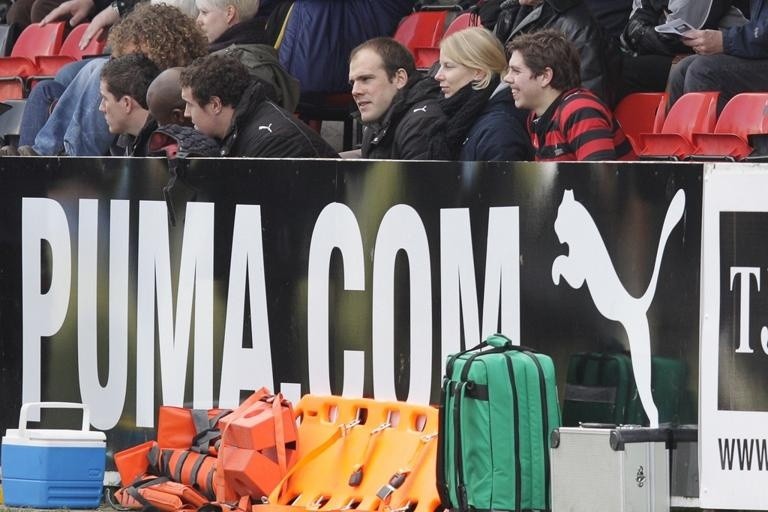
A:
435,26,535,160
1,0,415,160
466,1,768,123
503,29,640,160
349,38,446,160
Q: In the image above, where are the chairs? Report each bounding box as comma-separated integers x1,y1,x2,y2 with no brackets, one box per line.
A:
615,89,767,159
0,23,105,104
386,2,481,73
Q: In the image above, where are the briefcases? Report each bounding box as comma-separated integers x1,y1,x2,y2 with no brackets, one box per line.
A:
551,422,673,512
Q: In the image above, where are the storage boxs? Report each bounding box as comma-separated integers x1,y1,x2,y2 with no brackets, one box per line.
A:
0,401,108,509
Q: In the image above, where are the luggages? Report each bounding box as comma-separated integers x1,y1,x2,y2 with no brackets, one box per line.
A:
565,339,687,425
436,333,563,512
